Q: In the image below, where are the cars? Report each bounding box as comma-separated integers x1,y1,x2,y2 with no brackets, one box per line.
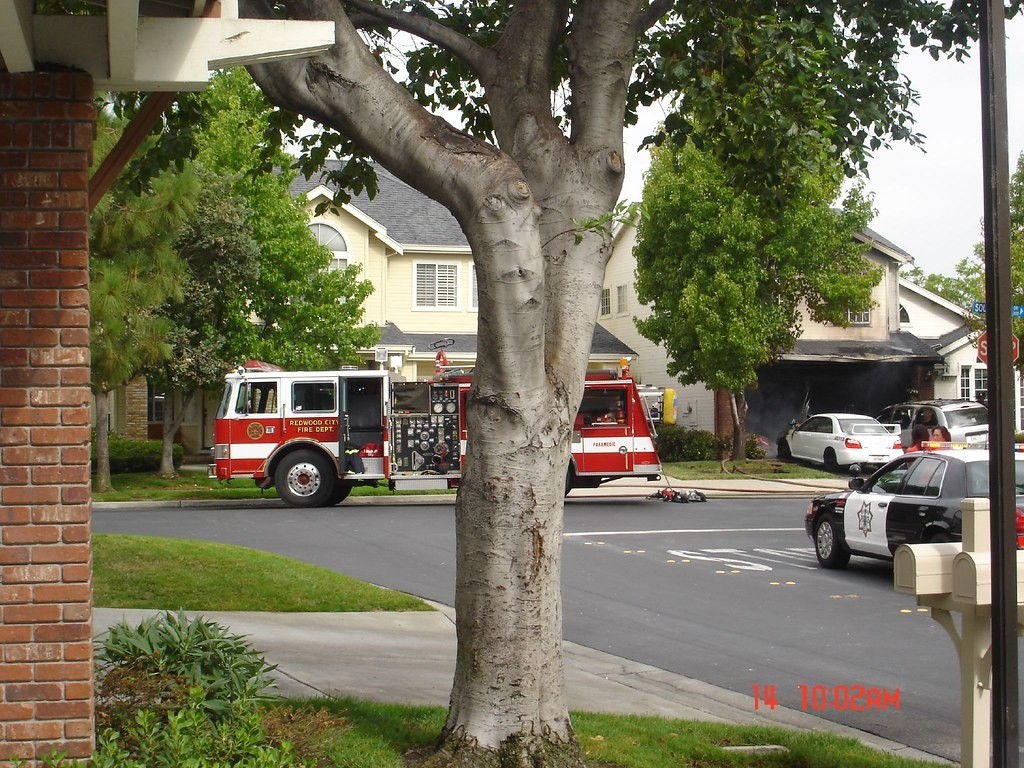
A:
804,440,1024,571
776,412,904,473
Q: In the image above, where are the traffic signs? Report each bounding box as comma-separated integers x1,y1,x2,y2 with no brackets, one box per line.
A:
972,301,1024,317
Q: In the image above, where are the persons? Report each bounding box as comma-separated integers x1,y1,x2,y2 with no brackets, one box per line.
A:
904,424,930,470
932,425,952,443
975,391,988,409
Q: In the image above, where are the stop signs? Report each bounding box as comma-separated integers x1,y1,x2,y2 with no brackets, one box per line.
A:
976,327,1019,367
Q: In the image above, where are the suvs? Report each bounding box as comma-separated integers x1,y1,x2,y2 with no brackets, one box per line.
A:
875,397,990,452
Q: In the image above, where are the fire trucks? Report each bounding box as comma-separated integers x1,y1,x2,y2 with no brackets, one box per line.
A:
206,337,680,509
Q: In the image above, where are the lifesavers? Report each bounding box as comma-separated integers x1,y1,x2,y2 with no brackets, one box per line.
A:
435,351,448,373
